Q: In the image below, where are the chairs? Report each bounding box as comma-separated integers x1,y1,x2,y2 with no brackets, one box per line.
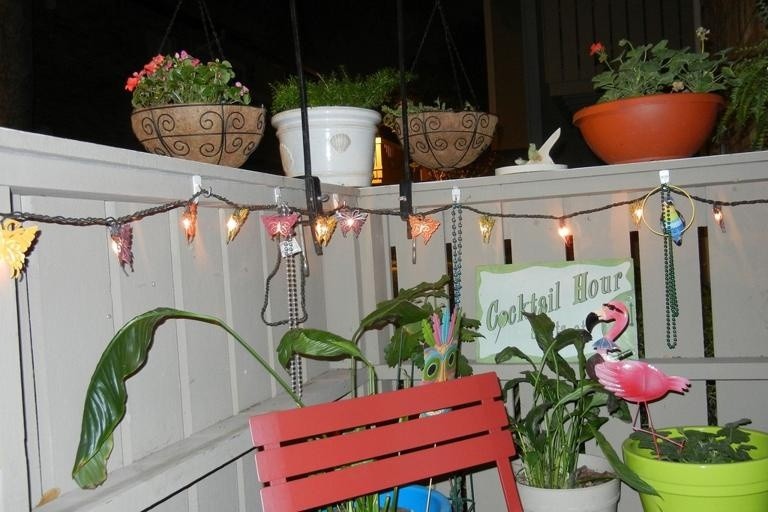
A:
247,370,524,512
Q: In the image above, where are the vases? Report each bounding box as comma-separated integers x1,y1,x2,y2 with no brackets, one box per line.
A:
571,92,726,165
129,105,268,168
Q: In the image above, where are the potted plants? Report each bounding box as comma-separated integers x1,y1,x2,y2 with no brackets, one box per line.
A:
71,270,488,512
267,64,419,189
494,312,665,512
380,96,501,174
621,417,768,512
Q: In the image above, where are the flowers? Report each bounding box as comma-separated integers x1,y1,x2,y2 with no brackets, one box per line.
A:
588,25,754,105
124,49,252,111
